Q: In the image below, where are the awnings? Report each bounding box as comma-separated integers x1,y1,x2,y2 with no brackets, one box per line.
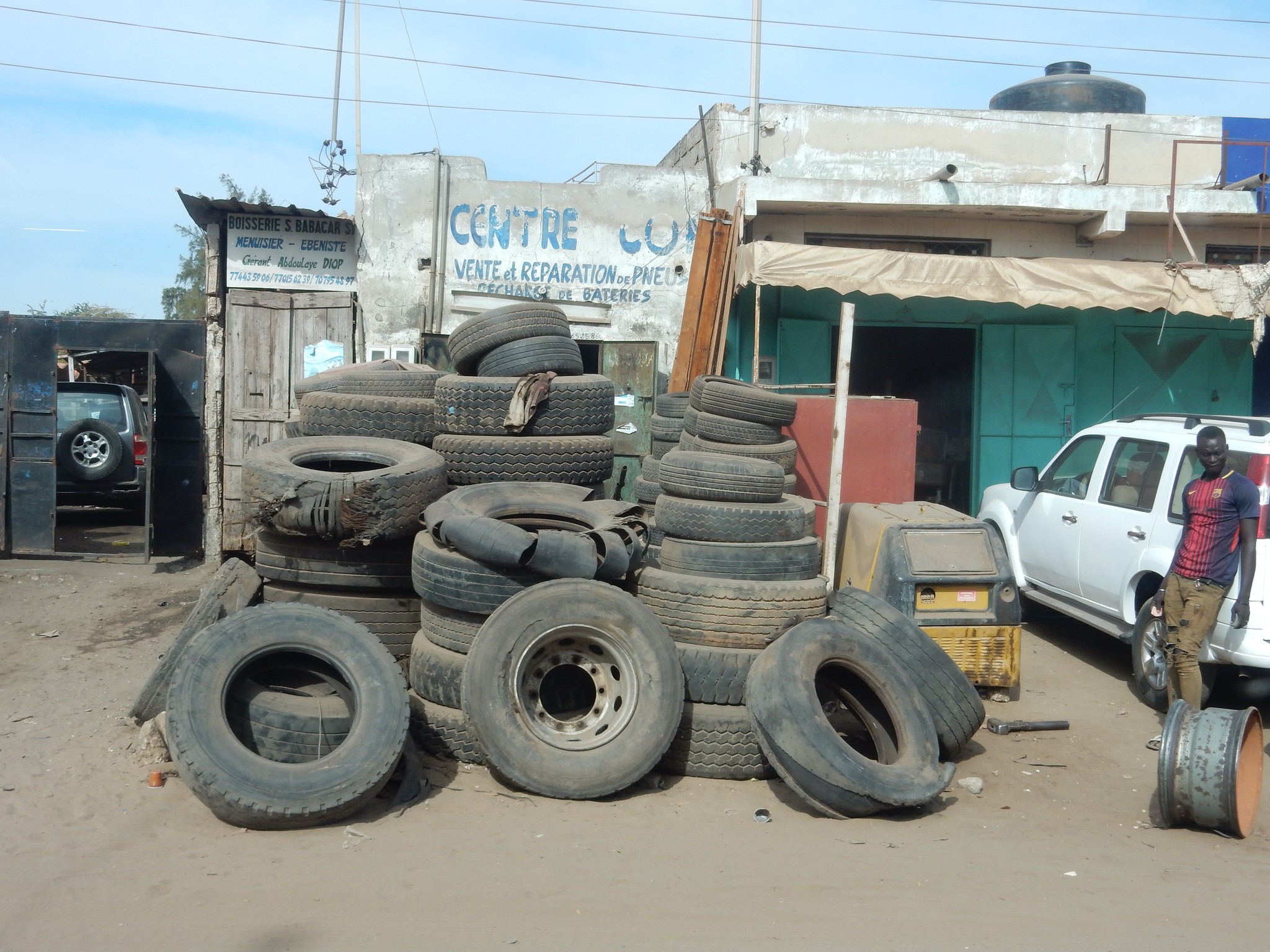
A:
730,240,1270,355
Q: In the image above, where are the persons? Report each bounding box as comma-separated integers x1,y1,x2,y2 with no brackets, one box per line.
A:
1142,426,1261,750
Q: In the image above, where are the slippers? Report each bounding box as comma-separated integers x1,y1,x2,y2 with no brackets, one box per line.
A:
1146,735,1162,750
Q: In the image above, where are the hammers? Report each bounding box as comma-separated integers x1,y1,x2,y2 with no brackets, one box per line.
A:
986,718,1069,735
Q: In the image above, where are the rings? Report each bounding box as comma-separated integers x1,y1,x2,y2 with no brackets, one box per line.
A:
1245,621,1248,622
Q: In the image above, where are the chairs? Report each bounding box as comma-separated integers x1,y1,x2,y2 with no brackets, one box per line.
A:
946,436,971,498
915,427,949,492
1111,452,1165,508
99,409,123,432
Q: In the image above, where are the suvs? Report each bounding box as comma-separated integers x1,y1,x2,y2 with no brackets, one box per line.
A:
54,381,148,511
973,412,1270,714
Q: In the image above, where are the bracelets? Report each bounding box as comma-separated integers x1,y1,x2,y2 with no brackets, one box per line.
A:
1236,599,1249,605
1157,589,1166,592
